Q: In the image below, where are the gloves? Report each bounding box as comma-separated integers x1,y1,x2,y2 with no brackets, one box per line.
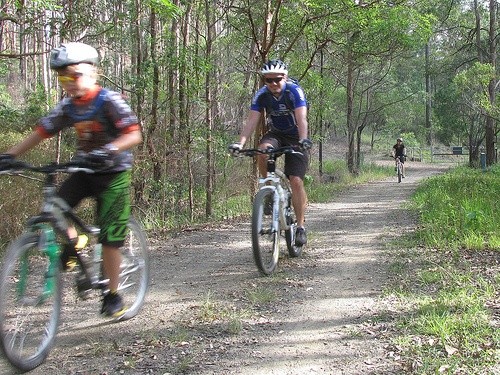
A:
84,148,110,169
1,153,14,160
228,143,242,155
299,139,313,149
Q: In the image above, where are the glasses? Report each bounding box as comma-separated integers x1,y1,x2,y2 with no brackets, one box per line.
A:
59,71,83,81
265,76,283,83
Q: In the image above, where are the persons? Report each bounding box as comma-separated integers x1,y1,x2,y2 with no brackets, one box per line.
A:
392,138,407,178
0,42,141,317
228,60,308,244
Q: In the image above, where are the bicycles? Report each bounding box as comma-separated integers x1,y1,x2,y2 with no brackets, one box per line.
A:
390,155,408,183
0,160,149,371
235,145,305,275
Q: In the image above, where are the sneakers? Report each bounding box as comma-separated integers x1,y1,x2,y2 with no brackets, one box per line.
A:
264,201,273,215
62,234,88,272
105,293,126,319
296,227,307,245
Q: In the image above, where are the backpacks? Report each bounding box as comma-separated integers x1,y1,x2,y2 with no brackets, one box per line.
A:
285,77,298,109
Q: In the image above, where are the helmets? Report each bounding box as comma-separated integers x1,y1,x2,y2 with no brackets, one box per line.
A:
261,60,288,75
50,41,98,70
397,138,402,141
